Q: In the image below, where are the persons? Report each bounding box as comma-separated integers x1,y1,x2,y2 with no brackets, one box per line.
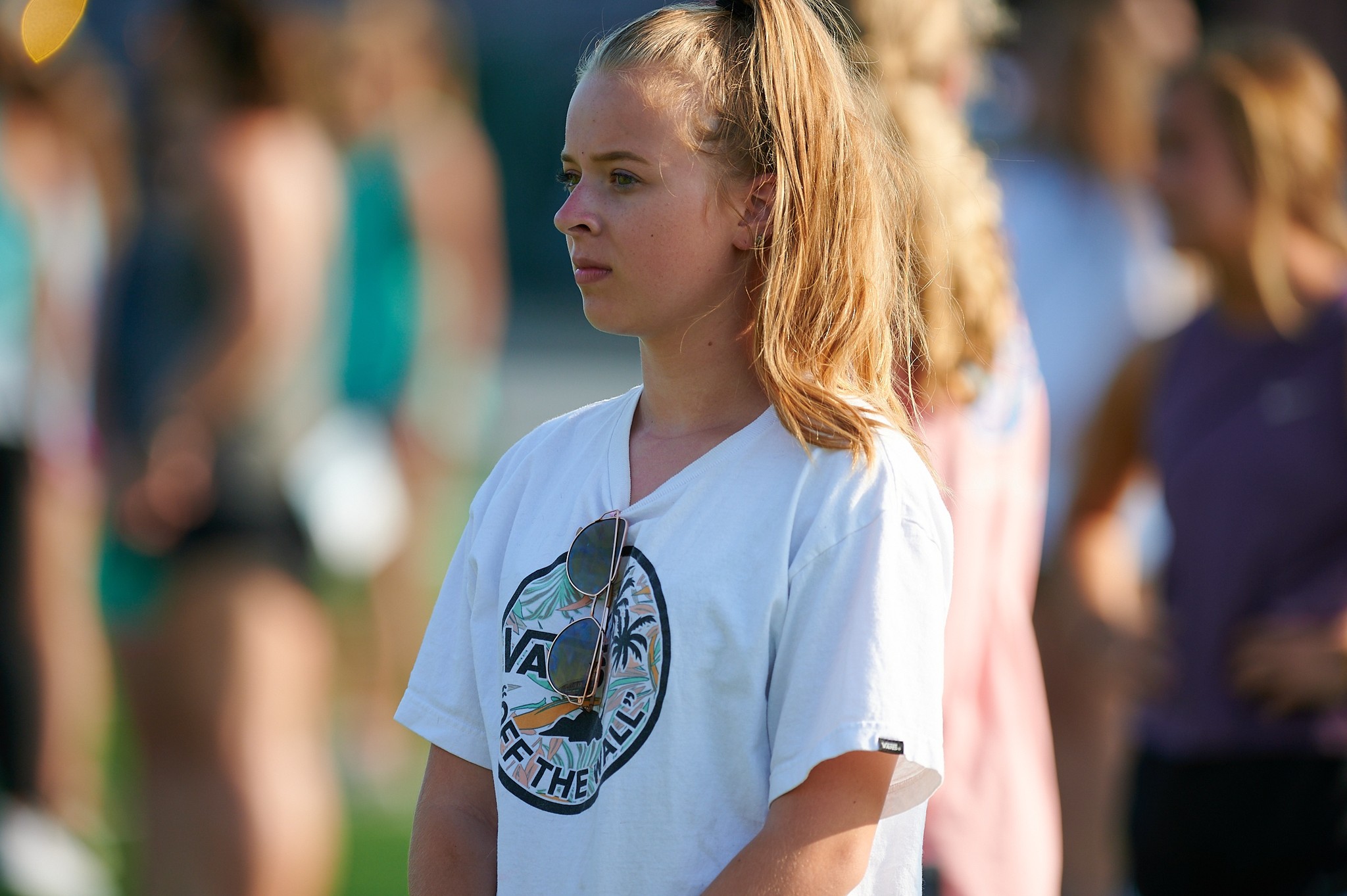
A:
395,0,955,896
851,0,1347,896
0,0,505,896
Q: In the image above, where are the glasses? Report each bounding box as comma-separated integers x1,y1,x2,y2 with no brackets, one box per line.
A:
545,509,629,714
